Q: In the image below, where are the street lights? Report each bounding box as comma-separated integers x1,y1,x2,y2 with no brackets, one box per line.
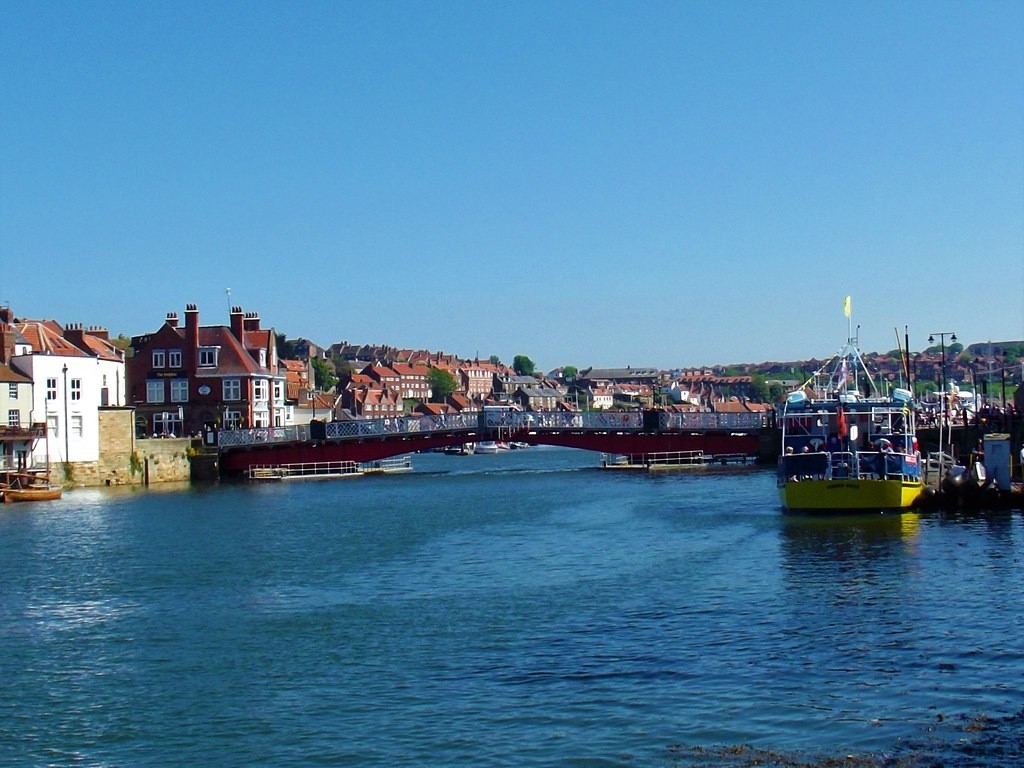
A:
927,333,957,422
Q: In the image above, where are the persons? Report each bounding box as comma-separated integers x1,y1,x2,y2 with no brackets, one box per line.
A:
136,430,202,439
914,403,1015,428
873,426,884,435
526,405,551,426
766,406,777,428
219,427,265,444
457,410,467,428
784,438,829,482
436,409,447,429
858,441,909,481
384,415,400,433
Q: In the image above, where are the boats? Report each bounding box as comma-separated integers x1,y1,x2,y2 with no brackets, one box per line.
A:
0,472,64,503
776,294,930,512
363,454,415,474
415,440,530,455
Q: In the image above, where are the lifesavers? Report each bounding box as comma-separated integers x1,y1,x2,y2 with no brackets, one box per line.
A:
872,438,894,452
806,437,826,452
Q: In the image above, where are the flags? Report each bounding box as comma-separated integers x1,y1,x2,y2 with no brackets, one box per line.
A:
837,359,847,389
844,296,851,318
